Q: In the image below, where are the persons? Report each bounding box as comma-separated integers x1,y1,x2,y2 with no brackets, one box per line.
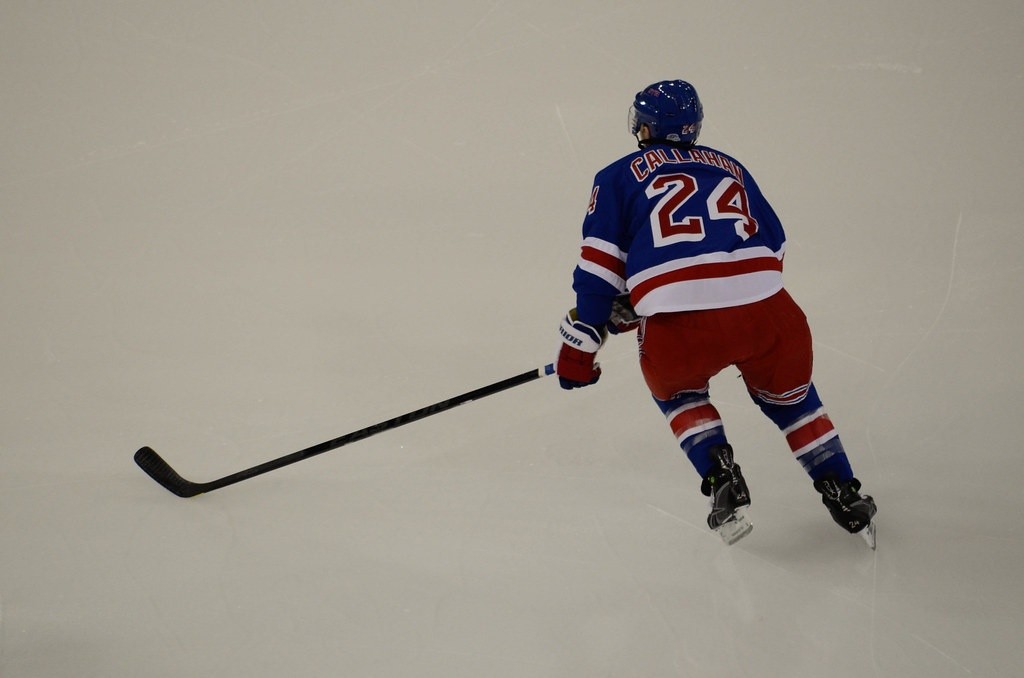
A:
556,79,877,552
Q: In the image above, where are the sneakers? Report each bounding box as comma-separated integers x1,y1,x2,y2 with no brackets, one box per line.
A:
812,470,879,551
701,441,752,546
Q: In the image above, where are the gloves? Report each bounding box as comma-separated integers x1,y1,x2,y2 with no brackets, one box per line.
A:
605,308,646,333
557,307,608,392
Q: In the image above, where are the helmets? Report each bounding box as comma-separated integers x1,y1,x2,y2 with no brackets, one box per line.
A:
627,79,705,145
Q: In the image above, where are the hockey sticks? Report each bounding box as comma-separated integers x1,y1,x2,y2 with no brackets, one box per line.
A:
132,357,557,499
